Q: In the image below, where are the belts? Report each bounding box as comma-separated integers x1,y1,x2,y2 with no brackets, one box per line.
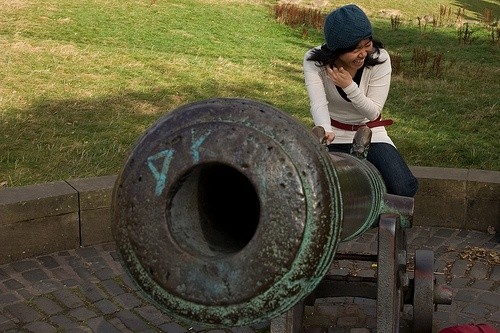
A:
331,113,394,131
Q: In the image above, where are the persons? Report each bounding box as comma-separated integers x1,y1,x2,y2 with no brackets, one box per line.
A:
302,3,420,199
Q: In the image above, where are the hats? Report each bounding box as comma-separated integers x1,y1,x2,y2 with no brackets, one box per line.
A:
325,4,373,52
